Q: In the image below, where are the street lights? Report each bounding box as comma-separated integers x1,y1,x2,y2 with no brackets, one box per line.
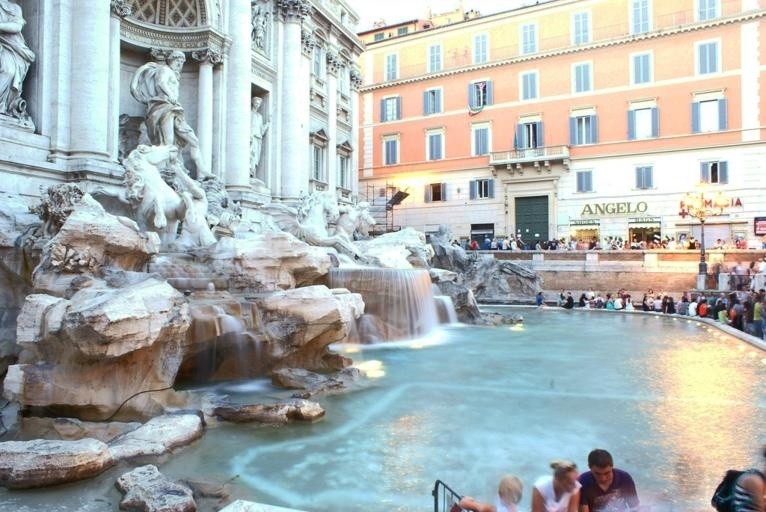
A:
683,181,732,291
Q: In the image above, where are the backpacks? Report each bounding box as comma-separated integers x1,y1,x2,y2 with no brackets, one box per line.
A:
711,469,764,512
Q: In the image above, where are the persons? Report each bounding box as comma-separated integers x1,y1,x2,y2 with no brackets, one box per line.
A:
716,236,748,250
449,232,602,250
576,448,642,512
252,5,267,47
535,291,549,307
710,443,765,512
532,459,584,512
604,232,701,250
127,42,221,182
458,474,524,511
0,0,37,116
554,258,766,343
246,92,271,180
